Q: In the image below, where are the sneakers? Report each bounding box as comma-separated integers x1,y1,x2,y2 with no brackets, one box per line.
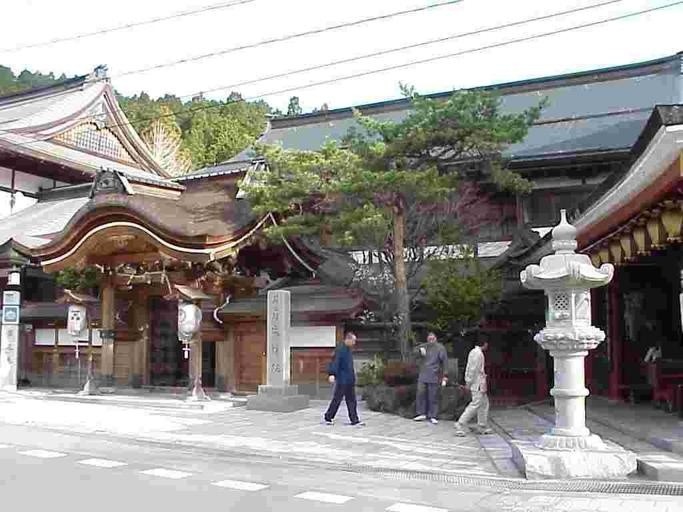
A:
453,423,465,437
478,427,494,435
349,421,368,428
321,412,335,425
413,414,439,424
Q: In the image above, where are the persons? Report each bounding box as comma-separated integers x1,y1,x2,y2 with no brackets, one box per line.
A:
410,331,448,423
453,333,494,436
321,330,362,425
640,341,662,383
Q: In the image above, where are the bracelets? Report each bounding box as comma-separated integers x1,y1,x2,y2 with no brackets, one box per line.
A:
441,378,448,382
